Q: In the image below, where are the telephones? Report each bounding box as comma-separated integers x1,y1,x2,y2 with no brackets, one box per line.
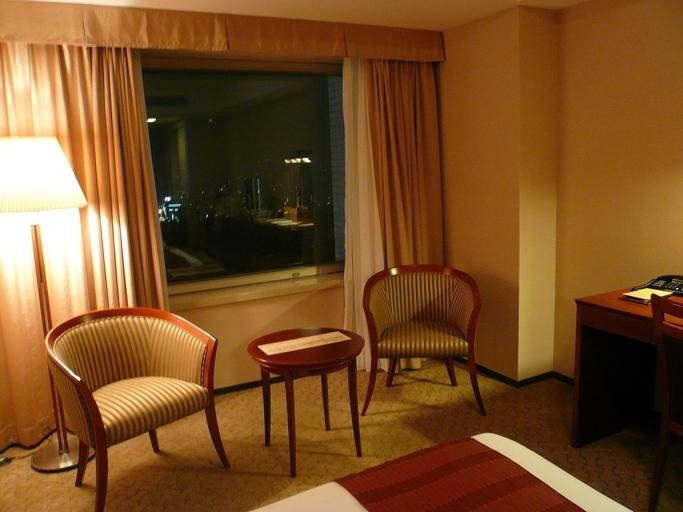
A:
651,274,683,297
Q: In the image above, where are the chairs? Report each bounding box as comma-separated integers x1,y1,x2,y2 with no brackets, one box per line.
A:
362,260,484,420
642,292,683,512
37,304,232,511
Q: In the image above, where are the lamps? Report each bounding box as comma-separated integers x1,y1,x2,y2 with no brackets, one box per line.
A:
0,133,103,474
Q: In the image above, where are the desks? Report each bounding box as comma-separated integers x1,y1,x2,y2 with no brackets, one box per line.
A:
572,283,682,441
247,325,367,481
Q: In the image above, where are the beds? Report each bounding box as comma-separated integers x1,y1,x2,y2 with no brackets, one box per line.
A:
249,432,637,512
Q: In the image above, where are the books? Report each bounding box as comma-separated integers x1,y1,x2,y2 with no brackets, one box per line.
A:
618,287,674,305
